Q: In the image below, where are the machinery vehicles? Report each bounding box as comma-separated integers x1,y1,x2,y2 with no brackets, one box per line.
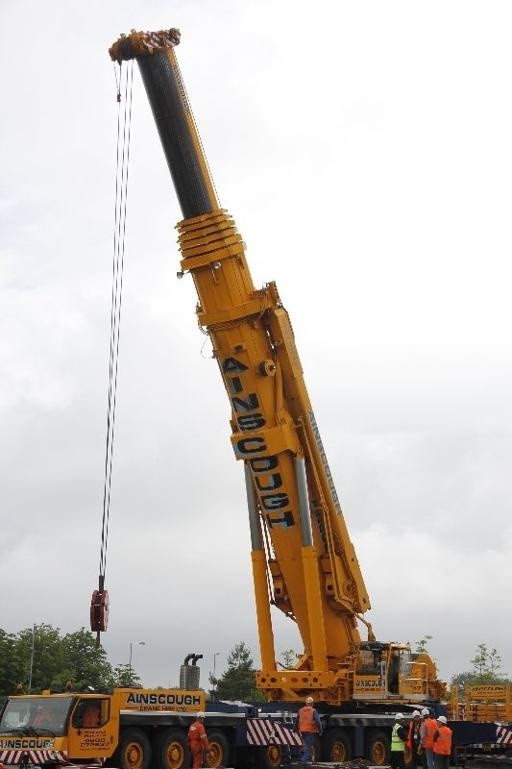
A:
2,25,512,769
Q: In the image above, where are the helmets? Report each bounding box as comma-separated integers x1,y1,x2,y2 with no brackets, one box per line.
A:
396,709,448,725
197,712,205,718
306,697,313,703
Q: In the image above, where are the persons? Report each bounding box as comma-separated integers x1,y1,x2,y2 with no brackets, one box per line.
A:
407,710,422,768
433,716,452,768
189,711,210,769
420,708,437,768
391,713,408,768
293,697,323,765
